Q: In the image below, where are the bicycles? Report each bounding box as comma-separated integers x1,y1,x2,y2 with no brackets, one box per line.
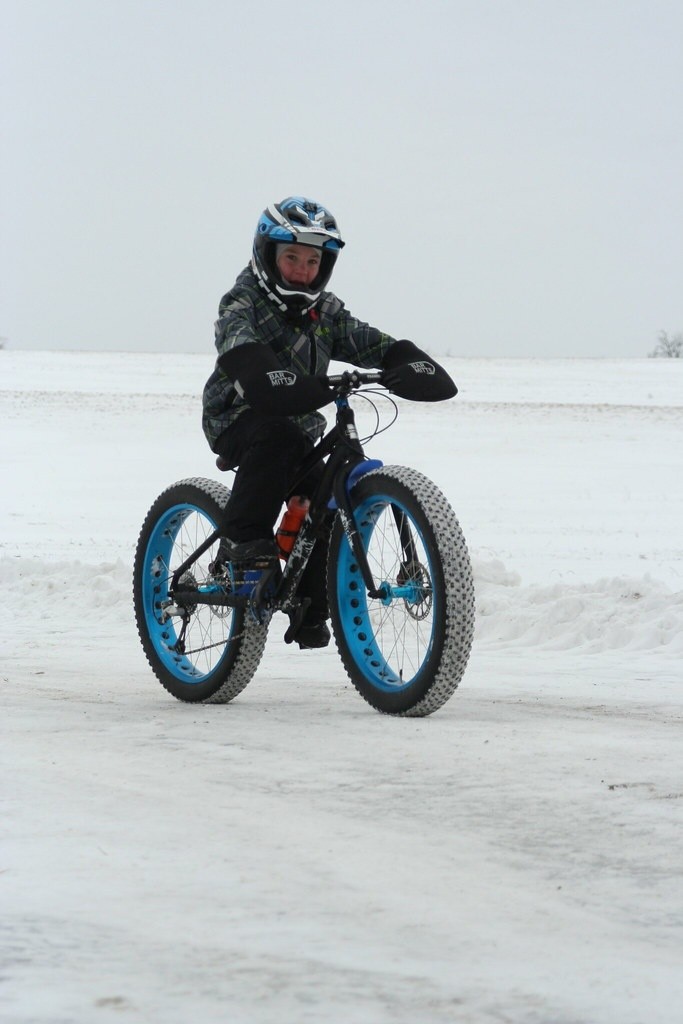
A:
132,370,477,719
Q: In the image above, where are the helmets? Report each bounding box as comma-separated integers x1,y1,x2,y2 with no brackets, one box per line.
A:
252,196,345,319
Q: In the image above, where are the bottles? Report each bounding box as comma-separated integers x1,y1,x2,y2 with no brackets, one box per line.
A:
276,496,309,559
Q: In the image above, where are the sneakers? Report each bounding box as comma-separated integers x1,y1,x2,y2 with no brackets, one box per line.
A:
216,527,278,569
289,588,329,648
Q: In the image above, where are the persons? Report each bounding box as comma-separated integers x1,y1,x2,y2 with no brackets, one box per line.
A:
202,195,457,647
